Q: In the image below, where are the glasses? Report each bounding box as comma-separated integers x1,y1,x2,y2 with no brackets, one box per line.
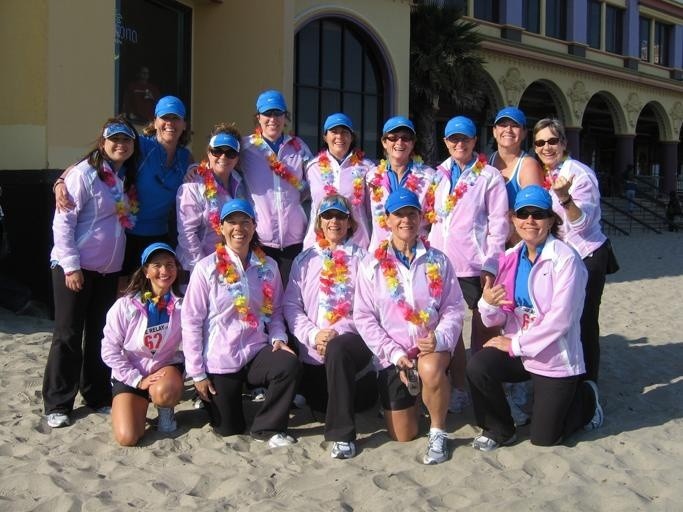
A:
264,111,283,116
321,211,347,220
517,209,547,220
386,135,411,141
534,138,559,146
211,148,236,158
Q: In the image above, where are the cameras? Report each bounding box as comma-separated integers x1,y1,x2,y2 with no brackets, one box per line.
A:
404,358,421,396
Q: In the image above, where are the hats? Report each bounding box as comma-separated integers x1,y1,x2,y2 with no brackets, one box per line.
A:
444,115,476,138
220,199,255,221
384,189,423,214
141,242,177,265
514,185,552,210
324,113,352,132
103,90,286,149
495,107,525,128
382,116,416,137
317,199,350,215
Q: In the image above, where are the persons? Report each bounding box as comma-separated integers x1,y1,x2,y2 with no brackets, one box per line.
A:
42,87,614,463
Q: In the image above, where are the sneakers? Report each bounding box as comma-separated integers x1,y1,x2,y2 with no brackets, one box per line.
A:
253,431,296,447
331,440,356,458
157,407,178,432
584,380,603,431
47,412,70,428
424,383,530,465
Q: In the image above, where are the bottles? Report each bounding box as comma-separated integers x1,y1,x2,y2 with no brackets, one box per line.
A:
407,358,420,397
